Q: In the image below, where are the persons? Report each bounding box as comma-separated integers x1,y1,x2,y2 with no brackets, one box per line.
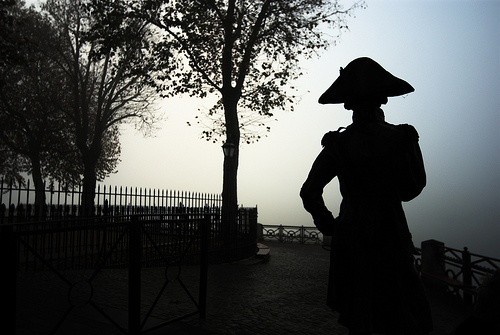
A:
299,57,433,335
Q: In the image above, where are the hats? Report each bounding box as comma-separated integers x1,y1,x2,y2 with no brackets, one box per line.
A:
315,56,414,106
